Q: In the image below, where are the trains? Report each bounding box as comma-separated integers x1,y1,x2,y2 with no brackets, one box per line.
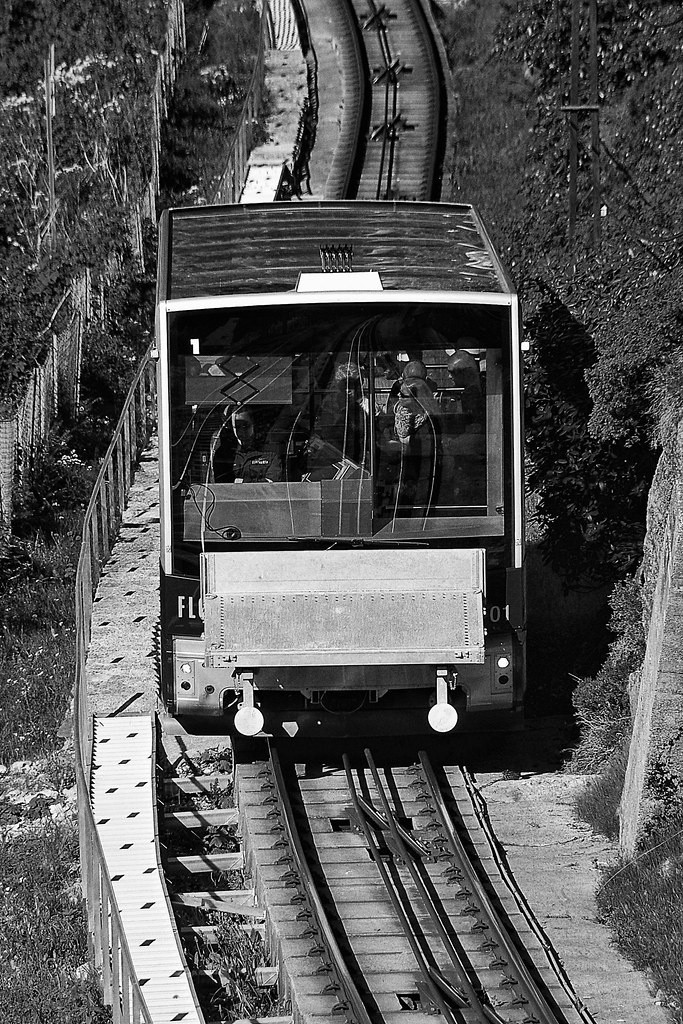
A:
154,200,530,737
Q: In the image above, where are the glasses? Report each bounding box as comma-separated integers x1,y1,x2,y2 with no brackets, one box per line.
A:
400,392,410,398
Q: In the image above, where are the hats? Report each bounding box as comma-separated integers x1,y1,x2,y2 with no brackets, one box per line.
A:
403,362,427,379
446,350,479,385
336,362,364,386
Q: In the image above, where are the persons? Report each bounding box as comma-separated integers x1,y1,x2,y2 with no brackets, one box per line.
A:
212,406,284,485
299,350,488,519
